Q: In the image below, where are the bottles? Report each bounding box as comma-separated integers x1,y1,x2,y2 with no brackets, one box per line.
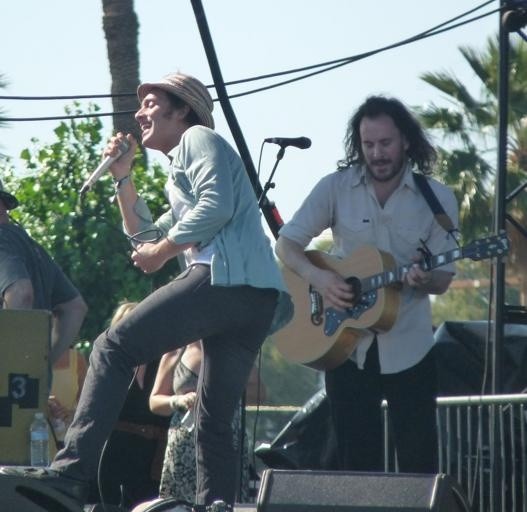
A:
29,413,50,467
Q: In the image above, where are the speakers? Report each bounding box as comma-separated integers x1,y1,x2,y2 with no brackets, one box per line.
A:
256,465,470,512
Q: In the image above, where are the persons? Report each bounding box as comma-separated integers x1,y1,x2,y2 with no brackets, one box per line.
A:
96,303,167,510
0,183,92,393
148,339,250,506
48,347,89,455
273,96,460,471
0,70,295,510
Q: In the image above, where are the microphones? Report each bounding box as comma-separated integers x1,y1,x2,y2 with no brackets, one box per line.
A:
262,136,314,149
79,137,132,195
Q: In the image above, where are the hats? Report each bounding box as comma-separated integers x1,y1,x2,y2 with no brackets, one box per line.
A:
137,72,213,127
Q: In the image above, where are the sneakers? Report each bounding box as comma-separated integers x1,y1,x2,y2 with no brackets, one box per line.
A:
1,465,90,508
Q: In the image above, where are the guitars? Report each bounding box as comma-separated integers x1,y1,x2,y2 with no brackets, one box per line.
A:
272,231,511,373
